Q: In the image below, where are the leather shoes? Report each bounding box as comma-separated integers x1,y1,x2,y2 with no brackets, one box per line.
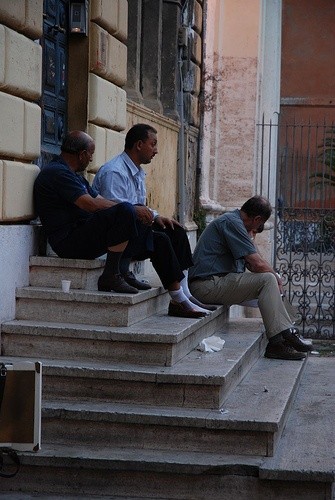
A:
167,295,217,318
98,271,153,293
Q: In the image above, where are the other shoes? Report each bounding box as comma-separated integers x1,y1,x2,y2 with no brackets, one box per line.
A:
264,327,312,360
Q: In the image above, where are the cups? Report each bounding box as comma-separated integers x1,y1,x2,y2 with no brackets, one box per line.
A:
62,280,71,292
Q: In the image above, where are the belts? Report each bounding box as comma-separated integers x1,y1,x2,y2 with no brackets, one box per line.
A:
189,275,202,284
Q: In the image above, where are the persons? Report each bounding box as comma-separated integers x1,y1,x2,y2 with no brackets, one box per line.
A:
187,196,314,361
90,123,217,318
34,129,154,293
234,222,312,346
